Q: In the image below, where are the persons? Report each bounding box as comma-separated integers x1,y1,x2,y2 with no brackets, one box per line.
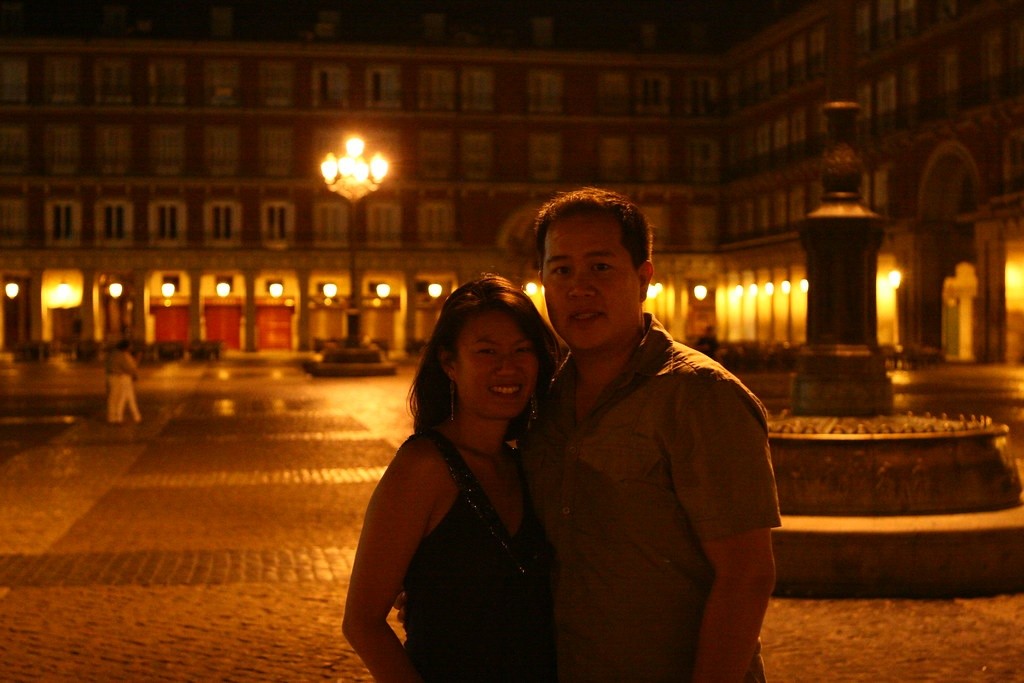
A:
393,187,777,683
106,339,145,427
343,276,559,683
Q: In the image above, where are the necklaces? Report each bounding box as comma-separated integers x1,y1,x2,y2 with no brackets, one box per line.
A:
419,431,529,577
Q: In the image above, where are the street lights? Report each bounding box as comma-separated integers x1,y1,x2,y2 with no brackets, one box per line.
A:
320,134,392,349
